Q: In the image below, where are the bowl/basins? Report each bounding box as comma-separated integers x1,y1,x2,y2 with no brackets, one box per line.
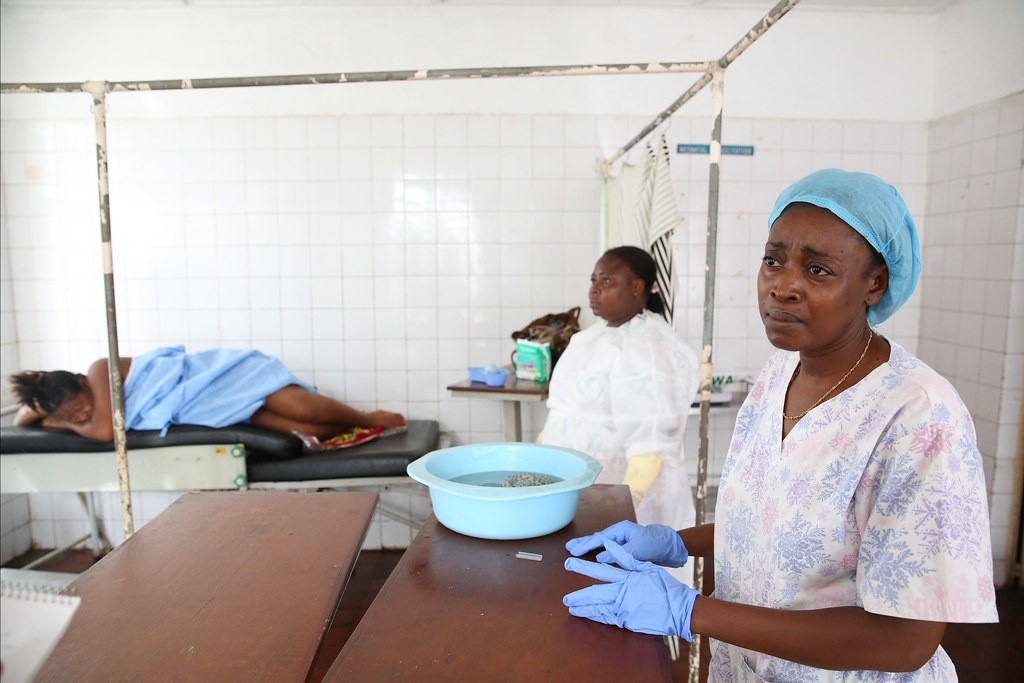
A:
404,443,601,538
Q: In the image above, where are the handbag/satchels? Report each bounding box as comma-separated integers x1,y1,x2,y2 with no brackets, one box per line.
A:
511,307,580,376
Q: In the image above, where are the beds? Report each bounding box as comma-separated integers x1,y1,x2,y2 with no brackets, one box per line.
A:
1,388,437,570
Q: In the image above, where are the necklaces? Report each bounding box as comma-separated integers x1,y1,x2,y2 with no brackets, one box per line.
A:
783,331,872,421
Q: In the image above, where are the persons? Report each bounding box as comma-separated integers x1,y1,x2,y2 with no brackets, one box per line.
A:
532,244,700,588
554,166,1000,683
10,345,407,444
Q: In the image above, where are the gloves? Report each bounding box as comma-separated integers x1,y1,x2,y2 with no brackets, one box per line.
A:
563,539,702,643
621,454,662,509
566,519,688,568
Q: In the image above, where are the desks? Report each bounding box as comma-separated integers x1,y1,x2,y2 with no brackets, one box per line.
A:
448,363,733,442
0,490,678,683
